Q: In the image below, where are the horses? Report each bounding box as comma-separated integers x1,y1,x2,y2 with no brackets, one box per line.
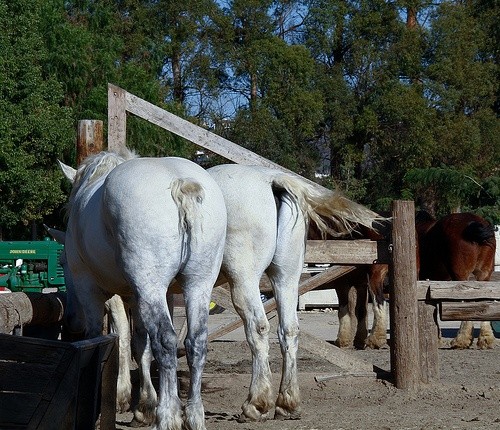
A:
309,211,497,350
57,143,228,430
106,164,392,427
